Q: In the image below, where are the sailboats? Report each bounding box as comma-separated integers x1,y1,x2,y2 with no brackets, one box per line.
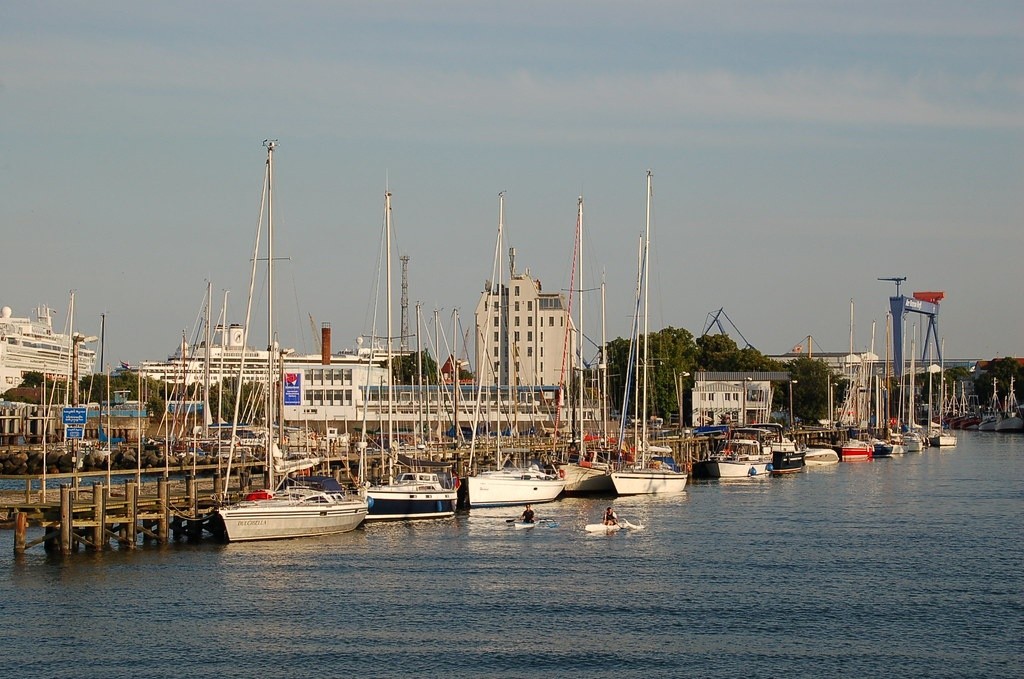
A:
11,133,1024,544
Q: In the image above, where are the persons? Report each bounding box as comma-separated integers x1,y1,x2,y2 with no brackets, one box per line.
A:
602,507,618,526
519,503,535,524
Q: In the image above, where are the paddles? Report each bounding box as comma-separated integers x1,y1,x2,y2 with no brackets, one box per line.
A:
504,518,550,523
